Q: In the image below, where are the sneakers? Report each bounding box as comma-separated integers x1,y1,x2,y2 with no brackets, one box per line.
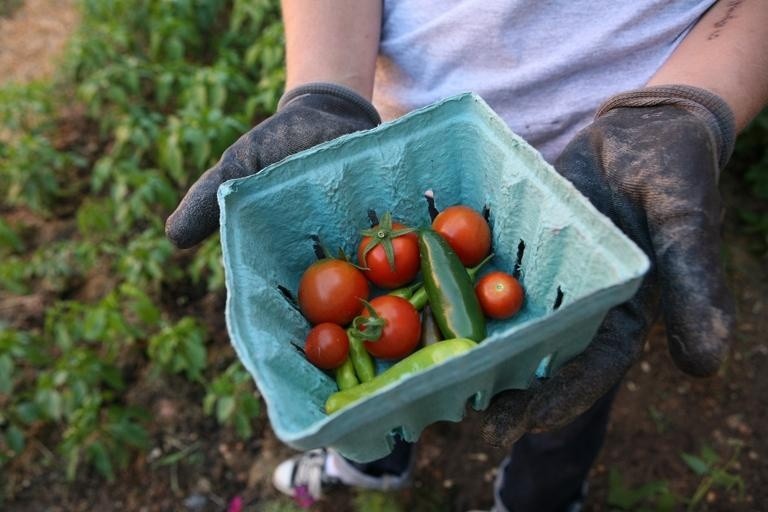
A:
272,447,417,502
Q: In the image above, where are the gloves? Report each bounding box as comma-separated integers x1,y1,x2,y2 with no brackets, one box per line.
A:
164,83,381,249
478,86,738,448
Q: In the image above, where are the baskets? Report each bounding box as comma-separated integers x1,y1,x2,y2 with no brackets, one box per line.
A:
217,91,651,462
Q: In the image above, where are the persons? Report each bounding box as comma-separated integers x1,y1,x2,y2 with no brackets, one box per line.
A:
165,0,768,512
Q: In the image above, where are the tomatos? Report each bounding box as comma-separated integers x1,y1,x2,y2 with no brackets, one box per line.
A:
298,203,522,368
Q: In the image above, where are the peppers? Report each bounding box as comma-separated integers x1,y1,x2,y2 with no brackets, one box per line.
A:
324,329,479,415
